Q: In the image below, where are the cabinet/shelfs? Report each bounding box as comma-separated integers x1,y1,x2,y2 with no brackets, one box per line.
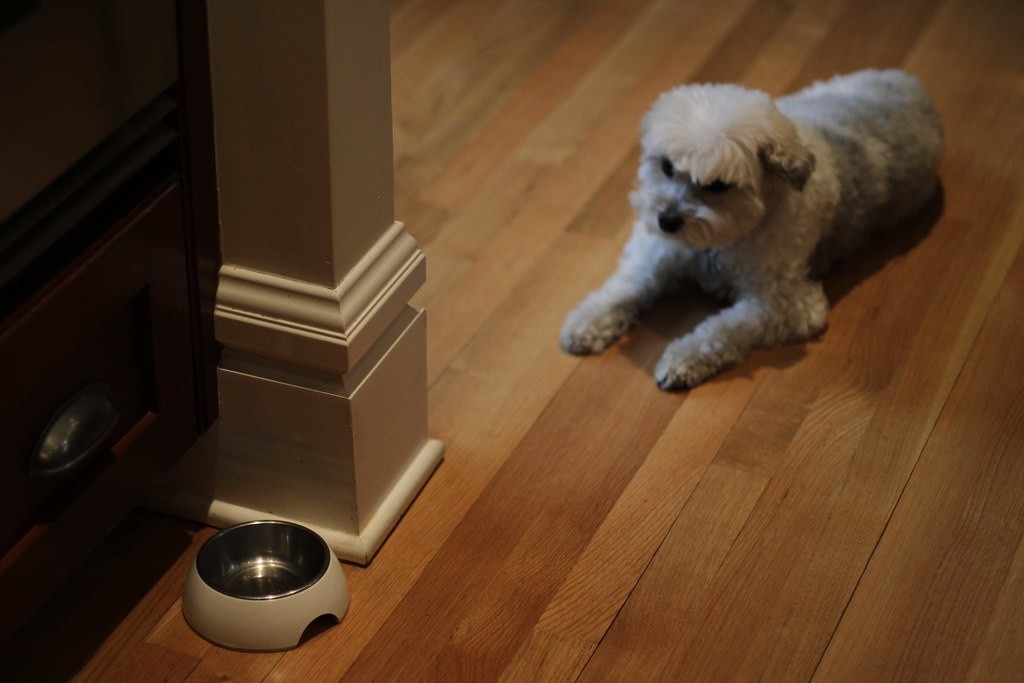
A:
0,0,221,651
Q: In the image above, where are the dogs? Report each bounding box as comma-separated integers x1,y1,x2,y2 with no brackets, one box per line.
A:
557,67,945,391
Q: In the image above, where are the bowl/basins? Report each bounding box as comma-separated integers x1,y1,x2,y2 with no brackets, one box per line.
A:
27,380,123,477
181,519,351,651
